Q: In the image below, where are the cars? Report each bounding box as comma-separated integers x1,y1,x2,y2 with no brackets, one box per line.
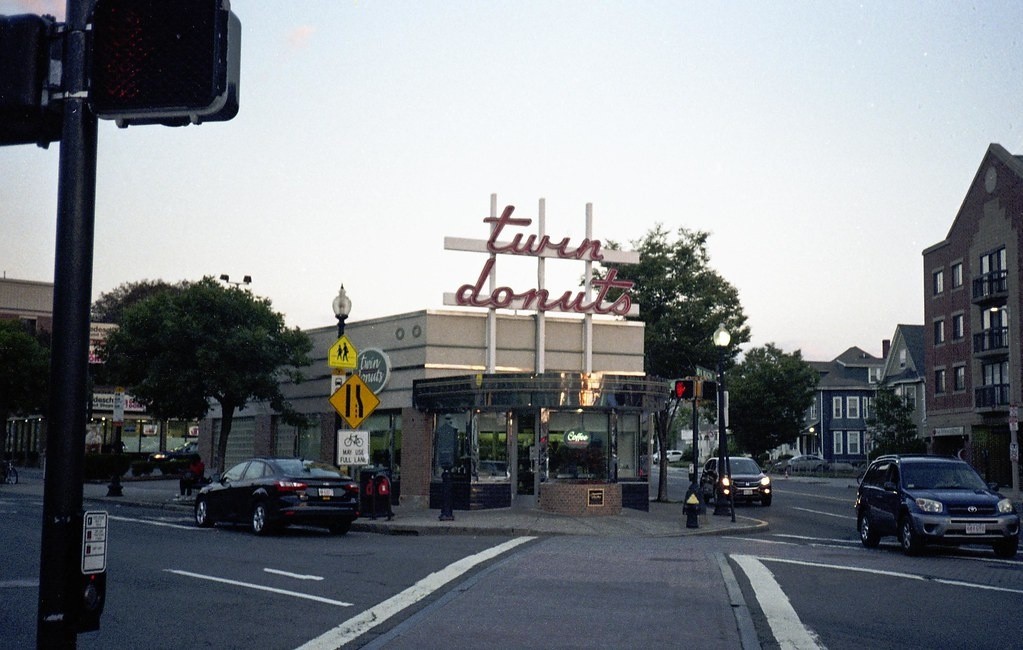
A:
652,449,683,464
194,455,359,536
775,454,854,475
149,440,199,477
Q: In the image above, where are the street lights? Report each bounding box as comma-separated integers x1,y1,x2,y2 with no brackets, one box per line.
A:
331,282,351,472
712,322,732,515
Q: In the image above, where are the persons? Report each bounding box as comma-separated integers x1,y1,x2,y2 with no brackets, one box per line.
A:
179,454,205,501
935,469,960,488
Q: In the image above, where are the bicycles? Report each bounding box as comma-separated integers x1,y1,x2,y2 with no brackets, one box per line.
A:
2,458,18,485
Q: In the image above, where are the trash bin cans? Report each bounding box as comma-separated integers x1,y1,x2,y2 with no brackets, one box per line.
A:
360,467,395,518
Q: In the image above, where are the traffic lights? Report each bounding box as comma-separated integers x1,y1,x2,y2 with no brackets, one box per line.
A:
84,0,241,129
675,379,697,400
697,378,717,403
0,12,64,153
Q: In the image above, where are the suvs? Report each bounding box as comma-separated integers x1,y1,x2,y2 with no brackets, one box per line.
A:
855,453,1021,559
700,457,772,507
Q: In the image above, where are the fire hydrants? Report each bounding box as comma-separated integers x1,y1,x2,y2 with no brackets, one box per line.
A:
684,493,701,529
